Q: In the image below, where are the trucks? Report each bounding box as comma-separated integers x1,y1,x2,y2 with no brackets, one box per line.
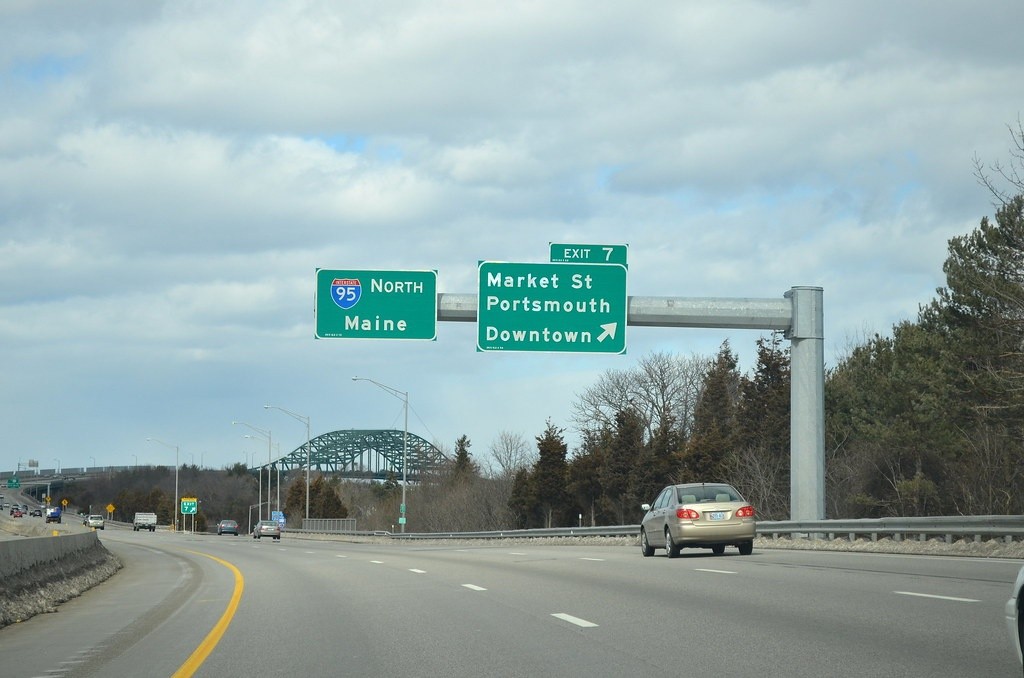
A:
132,512,157,531
44,506,61,524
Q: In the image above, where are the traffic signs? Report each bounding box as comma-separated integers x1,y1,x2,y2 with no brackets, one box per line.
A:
477,244,630,357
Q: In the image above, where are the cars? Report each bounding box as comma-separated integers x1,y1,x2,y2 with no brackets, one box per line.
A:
4,502,9,507
32,509,42,517
9,504,34,518
639,481,756,559
88,514,105,531
0,505,3,510
0,494,4,499
216,519,238,536
253,519,281,539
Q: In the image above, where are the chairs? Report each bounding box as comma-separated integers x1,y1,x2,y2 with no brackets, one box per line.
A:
716,494,730,502
682,495,697,504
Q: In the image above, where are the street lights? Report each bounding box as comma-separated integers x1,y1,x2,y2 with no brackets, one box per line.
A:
231,421,272,522
264,404,312,521
351,376,405,534
146,437,179,531
53,457,62,476
89,456,96,469
248,501,272,535
132,454,138,467
244,436,281,512
